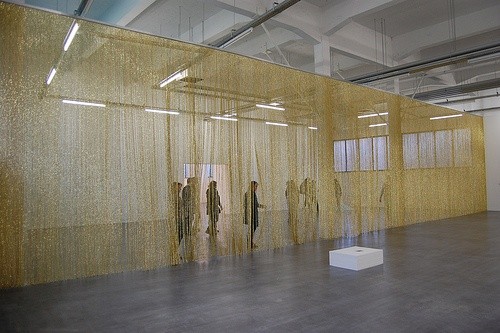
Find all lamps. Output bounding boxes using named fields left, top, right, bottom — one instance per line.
left=159, top=68, right=183, bottom=88
left=61, top=18, right=80, bottom=51
left=46, top=65, right=57, bottom=85
left=62, top=98, right=107, bottom=108
left=255, top=103, right=285, bottom=111
left=145, top=106, right=180, bottom=115
left=218, top=27, right=253, bottom=49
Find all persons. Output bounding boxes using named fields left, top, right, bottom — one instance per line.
left=242, top=181, right=267, bottom=249
left=285, top=180, right=300, bottom=229
left=379, top=175, right=392, bottom=219
left=167, top=182, right=183, bottom=263
left=300, top=177, right=319, bottom=216
left=205, top=180, right=223, bottom=239
left=181, top=177, right=200, bottom=244
left=334, top=178, right=342, bottom=209
